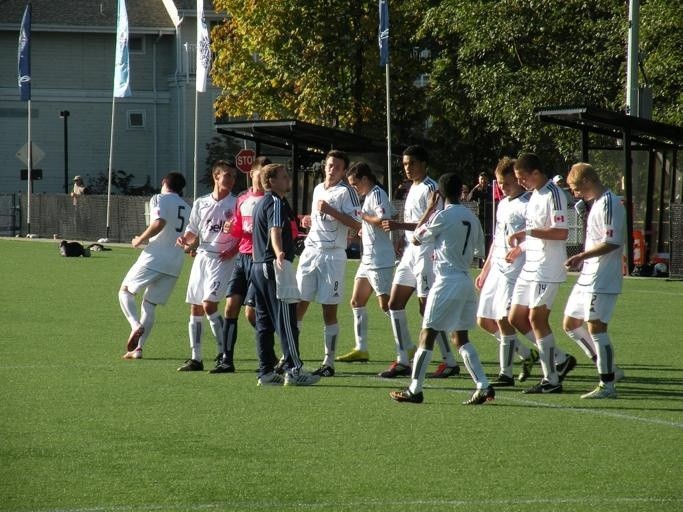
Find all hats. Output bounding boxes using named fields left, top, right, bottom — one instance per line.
left=73, top=176, right=81, bottom=181
left=553, top=175, right=564, bottom=183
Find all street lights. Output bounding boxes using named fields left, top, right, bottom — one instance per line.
left=58, top=109, right=72, bottom=195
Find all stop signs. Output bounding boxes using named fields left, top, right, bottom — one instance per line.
left=235, top=149, right=256, bottom=173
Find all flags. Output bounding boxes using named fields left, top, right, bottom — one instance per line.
left=377, top=1, right=391, bottom=67
left=16, top=4, right=34, bottom=103
left=113, top=0, right=133, bottom=99
left=194, top=1, right=212, bottom=94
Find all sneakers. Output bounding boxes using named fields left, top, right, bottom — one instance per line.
left=335, top=350, right=370, bottom=362
left=380, top=361, right=412, bottom=378
left=210, top=353, right=235, bottom=373
left=177, top=359, right=203, bottom=371
left=389, top=388, right=423, bottom=403
left=255, top=359, right=336, bottom=386
left=463, top=375, right=514, bottom=405
left=580, top=367, right=624, bottom=399
left=518, top=348, right=577, bottom=394
left=124, top=323, right=145, bottom=359
left=427, top=364, right=460, bottom=378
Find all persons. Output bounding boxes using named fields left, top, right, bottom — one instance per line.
left=70, top=174, right=88, bottom=228
left=561, top=163, right=627, bottom=400
left=504, top=153, right=577, bottom=394
left=334, top=161, right=418, bottom=363
left=176, top=158, right=241, bottom=371
left=475, top=158, right=540, bottom=390
left=210, top=156, right=298, bottom=373
left=379, top=145, right=460, bottom=378
left=390, top=174, right=495, bottom=405
left=117, top=171, right=191, bottom=361
left=461, top=171, right=493, bottom=222
left=58, top=240, right=91, bottom=258
left=253, top=163, right=322, bottom=387
left=272, top=150, right=361, bottom=375
